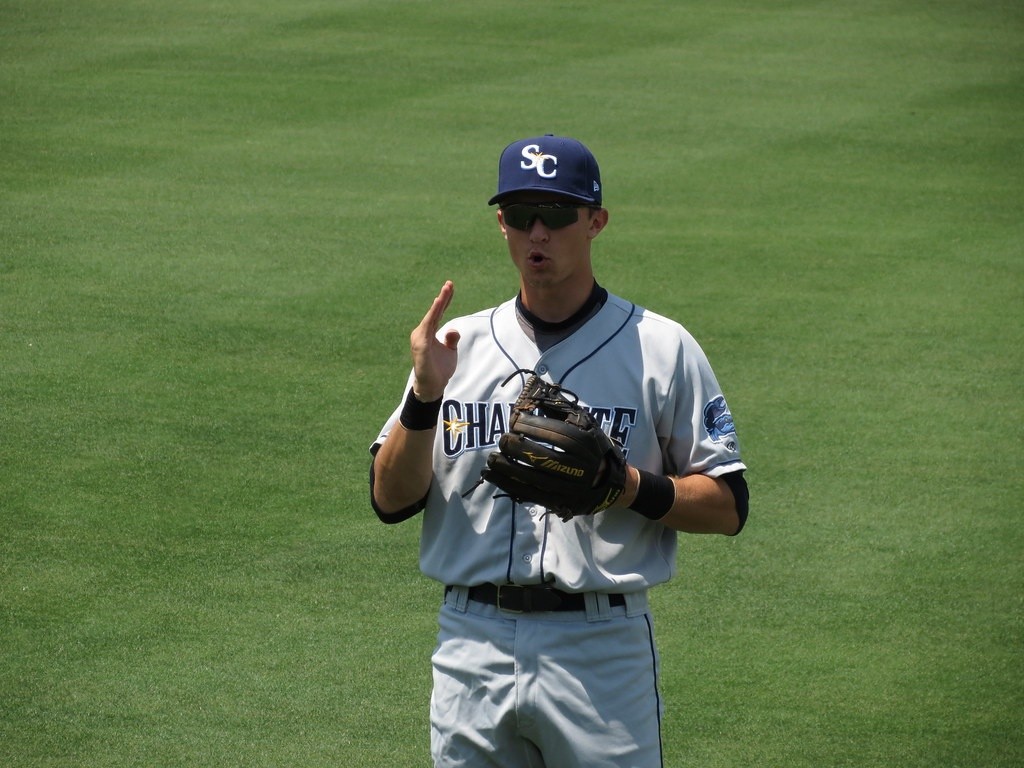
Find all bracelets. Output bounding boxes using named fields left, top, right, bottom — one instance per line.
left=628, top=468, right=676, bottom=522
left=399, top=386, right=444, bottom=431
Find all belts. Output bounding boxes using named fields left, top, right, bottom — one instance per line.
left=446, top=583, right=626, bottom=612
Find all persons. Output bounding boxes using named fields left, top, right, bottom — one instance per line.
left=371, top=136, right=748, bottom=768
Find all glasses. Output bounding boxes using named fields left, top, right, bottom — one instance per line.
left=500, top=200, right=593, bottom=231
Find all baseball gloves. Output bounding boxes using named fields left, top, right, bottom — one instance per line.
left=479, top=373, right=629, bottom=523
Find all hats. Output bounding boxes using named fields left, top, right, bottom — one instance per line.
left=487, top=133, right=602, bottom=207
